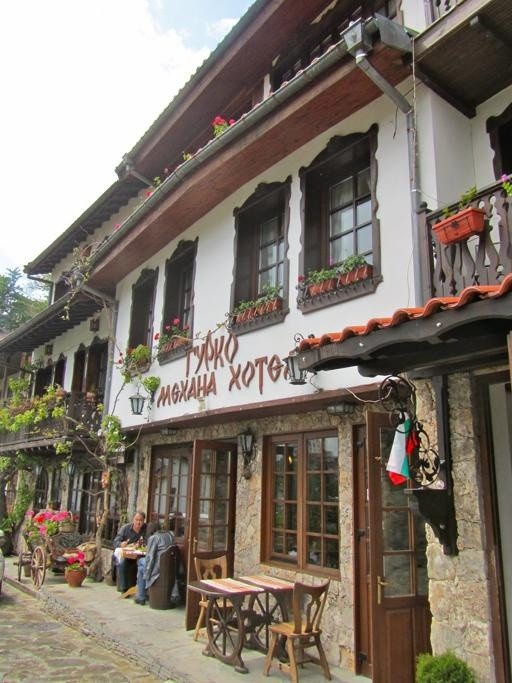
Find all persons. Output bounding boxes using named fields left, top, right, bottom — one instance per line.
left=134, top=522, right=172, bottom=605
left=113, top=513, right=149, bottom=594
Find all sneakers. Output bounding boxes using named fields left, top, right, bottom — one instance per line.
left=135, top=599, right=145, bottom=604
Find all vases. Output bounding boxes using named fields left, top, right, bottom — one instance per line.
left=157, top=335, right=188, bottom=355
left=65, top=569, right=87, bottom=587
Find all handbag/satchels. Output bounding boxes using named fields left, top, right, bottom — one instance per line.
left=170, top=582, right=182, bottom=605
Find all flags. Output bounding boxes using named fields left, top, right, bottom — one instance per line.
left=385, top=410, right=420, bottom=486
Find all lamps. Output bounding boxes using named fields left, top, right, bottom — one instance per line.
left=64, top=461, right=76, bottom=476
left=281, top=332, right=316, bottom=385
left=237, top=426, right=255, bottom=469
left=325, top=400, right=362, bottom=420
left=128, top=380, right=155, bottom=415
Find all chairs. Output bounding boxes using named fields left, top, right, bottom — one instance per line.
left=148, top=545, right=181, bottom=609
left=115, top=562, right=137, bottom=592
left=192, top=555, right=248, bottom=641
left=262, top=576, right=331, bottom=683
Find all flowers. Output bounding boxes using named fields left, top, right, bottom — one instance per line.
left=154, top=318, right=191, bottom=345
left=22, top=510, right=86, bottom=573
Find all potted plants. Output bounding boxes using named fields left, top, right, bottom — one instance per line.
left=431, top=185, right=486, bottom=245
left=304, top=255, right=372, bottom=297
left=231, top=285, right=283, bottom=325
